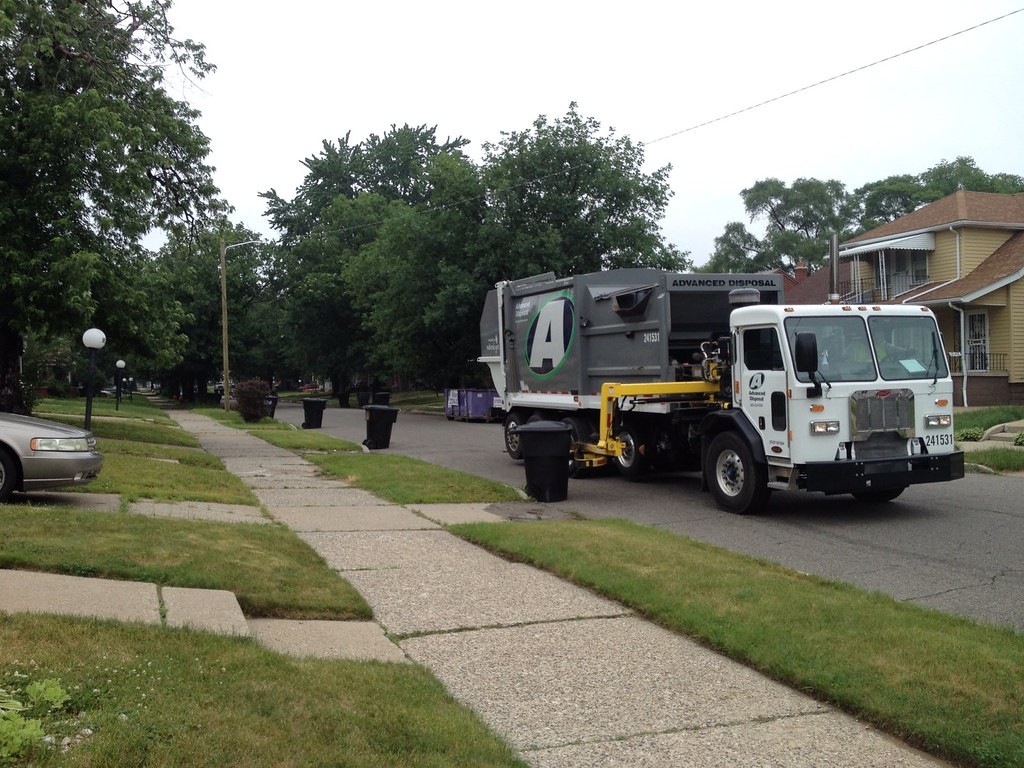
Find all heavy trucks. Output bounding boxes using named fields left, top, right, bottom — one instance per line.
left=476, top=268, right=965, bottom=517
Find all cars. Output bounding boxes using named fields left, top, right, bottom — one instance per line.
left=214, top=375, right=236, bottom=399
left=297, top=384, right=319, bottom=392
left=0, top=411, right=105, bottom=503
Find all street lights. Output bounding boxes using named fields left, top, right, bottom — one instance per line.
left=115, top=359, right=126, bottom=411
left=128, top=377, right=134, bottom=401
left=82, top=328, right=107, bottom=431
left=221, top=241, right=261, bottom=411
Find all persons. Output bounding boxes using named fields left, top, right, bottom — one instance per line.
left=847, top=318, right=912, bottom=363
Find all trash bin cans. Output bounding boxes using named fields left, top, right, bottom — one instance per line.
left=357, top=392, right=371, bottom=409
left=339, top=393, right=349, bottom=408
left=444, top=389, right=502, bottom=423
left=373, top=392, right=390, bottom=405
left=301, top=398, right=327, bottom=429
left=362, top=405, right=399, bottom=450
left=264, top=395, right=278, bottom=419
left=509, top=421, right=573, bottom=503
